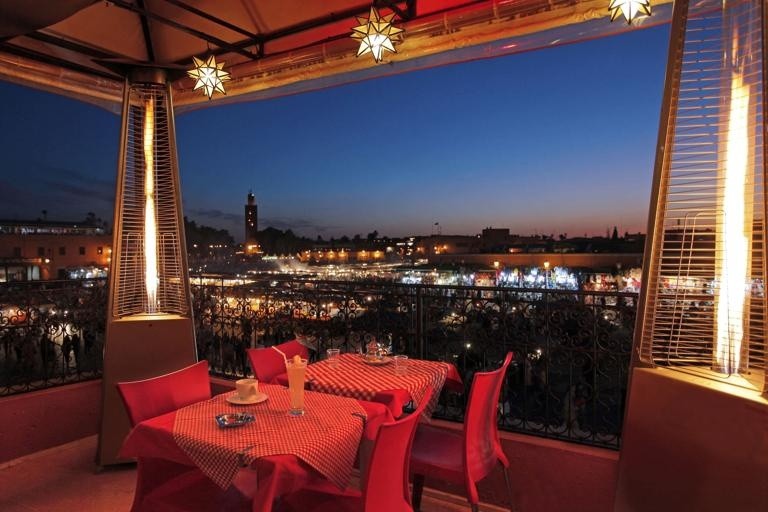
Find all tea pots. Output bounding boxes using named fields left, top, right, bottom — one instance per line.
left=366, top=338, right=388, bottom=362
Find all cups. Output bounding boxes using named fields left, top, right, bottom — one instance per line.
left=284, top=360, right=307, bottom=419
left=396, top=357, right=408, bottom=377
left=327, top=349, right=340, bottom=369
left=237, top=379, right=258, bottom=400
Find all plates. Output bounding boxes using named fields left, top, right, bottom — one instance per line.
left=362, top=356, right=393, bottom=366
left=226, top=393, right=268, bottom=405
left=216, top=413, right=255, bottom=427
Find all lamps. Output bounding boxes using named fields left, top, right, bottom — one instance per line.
left=87, top=60, right=198, bottom=468
left=350, top=7, right=405, bottom=63
left=185, top=55, right=232, bottom=99
left=608, top=1, right=652, bottom=23
left=614, top=0, right=767, bottom=511
